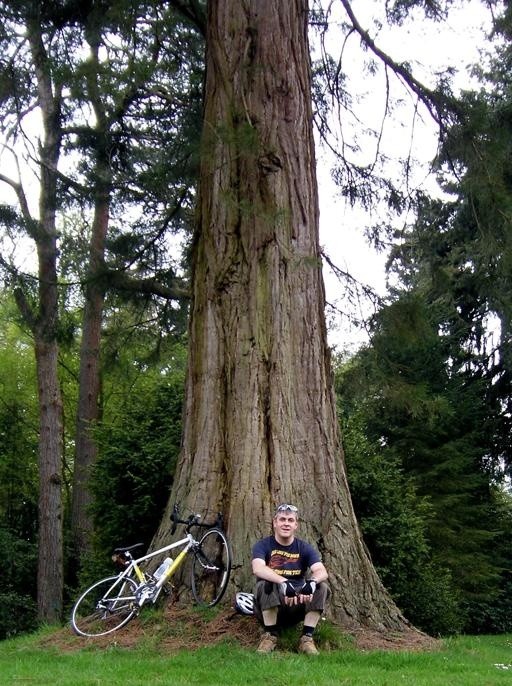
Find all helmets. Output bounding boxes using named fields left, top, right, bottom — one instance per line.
left=234, top=591, right=255, bottom=615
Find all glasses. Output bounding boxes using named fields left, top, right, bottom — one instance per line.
left=275, top=504, right=298, bottom=515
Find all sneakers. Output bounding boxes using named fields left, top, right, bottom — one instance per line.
left=256, top=632, right=277, bottom=653
left=297, top=635, right=319, bottom=655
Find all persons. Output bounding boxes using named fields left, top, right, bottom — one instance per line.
left=250, top=503, right=329, bottom=657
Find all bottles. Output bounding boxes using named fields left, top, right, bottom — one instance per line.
left=154, top=556, right=174, bottom=580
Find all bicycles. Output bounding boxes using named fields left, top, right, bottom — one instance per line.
left=72, top=512, right=234, bottom=640
